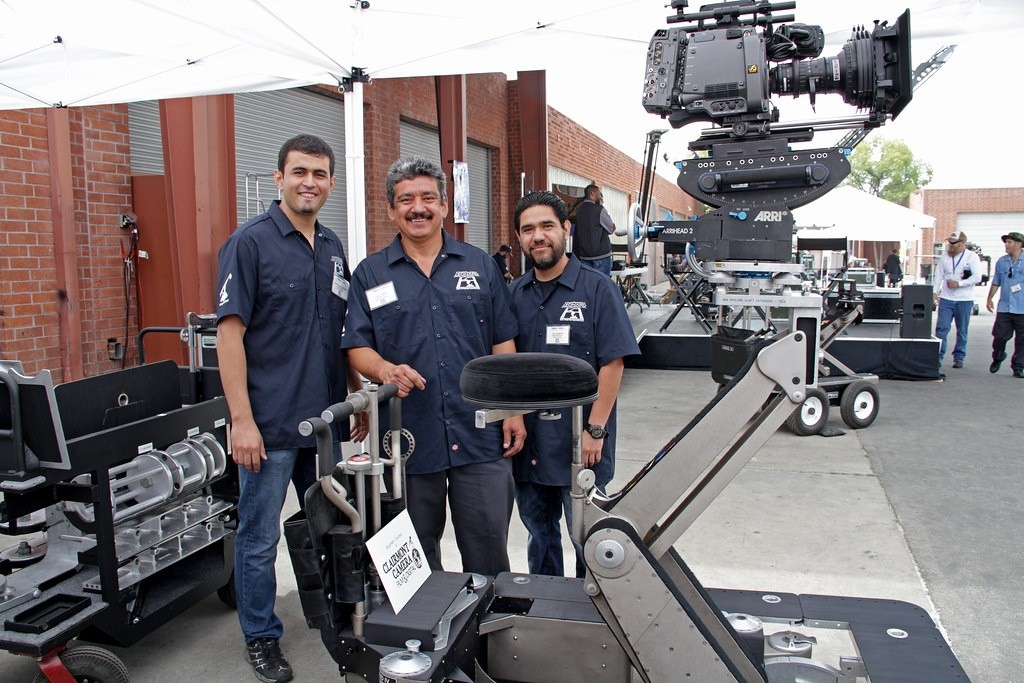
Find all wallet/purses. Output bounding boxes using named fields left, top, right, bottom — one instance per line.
left=961, top=269, right=972, bottom=280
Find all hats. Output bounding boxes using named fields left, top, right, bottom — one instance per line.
left=503, top=244, right=514, bottom=257
left=949, top=232, right=967, bottom=240
left=1002, top=232, right=1024, bottom=247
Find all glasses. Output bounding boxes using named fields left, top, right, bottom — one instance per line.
left=949, top=241, right=961, bottom=244
left=1008, top=267, right=1013, bottom=278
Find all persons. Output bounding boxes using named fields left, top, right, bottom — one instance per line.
left=342, top=157, right=526, bottom=575
left=215, top=133, right=368, bottom=683
left=887, top=249, right=900, bottom=288
left=506, top=189, right=643, bottom=578
left=569, top=184, right=616, bottom=278
left=492, top=244, right=514, bottom=282
left=986, top=233, right=1024, bottom=377
left=933, top=231, right=981, bottom=367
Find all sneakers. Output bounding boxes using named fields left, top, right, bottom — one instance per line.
left=953, top=361, right=963, bottom=368
left=990, top=353, right=1007, bottom=373
left=1013, top=369, right=1024, bottom=378
left=244, top=637, right=293, bottom=683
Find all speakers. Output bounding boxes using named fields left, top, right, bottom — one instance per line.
left=900, top=285, right=933, bottom=339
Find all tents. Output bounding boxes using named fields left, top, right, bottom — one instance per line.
left=795, top=182, right=938, bottom=284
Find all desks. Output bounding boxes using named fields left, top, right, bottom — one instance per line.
left=611, top=266, right=649, bottom=312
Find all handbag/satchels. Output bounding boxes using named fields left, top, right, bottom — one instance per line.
left=883, top=262, right=888, bottom=273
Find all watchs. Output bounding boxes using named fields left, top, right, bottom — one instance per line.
left=586, top=423, right=609, bottom=440
